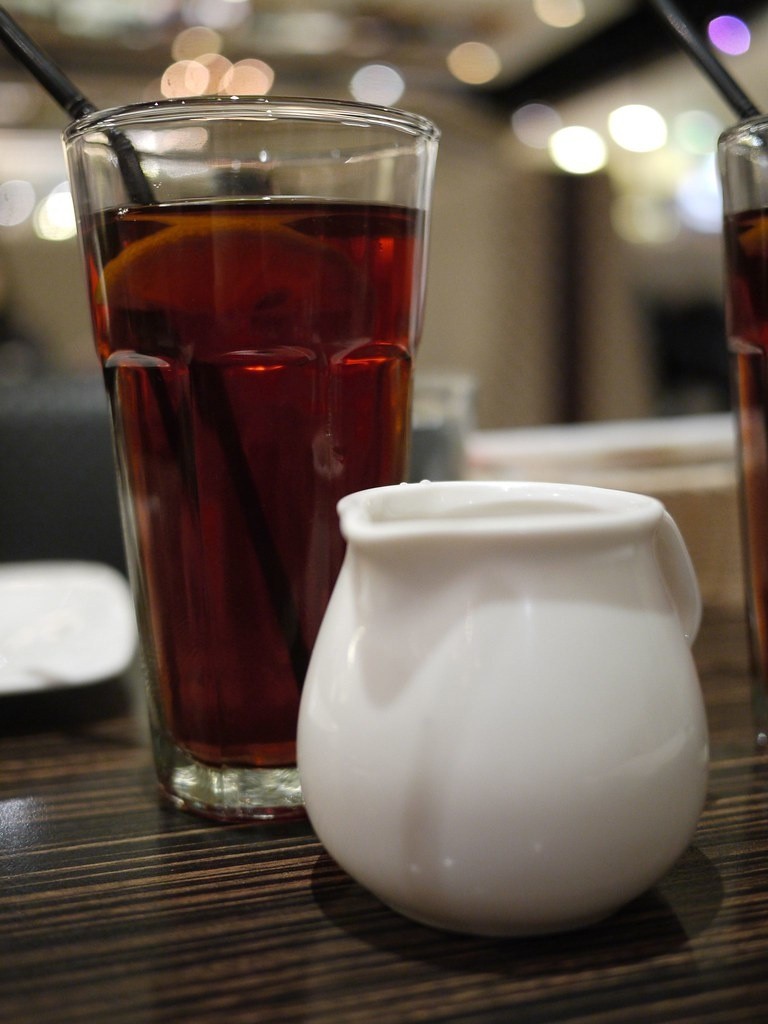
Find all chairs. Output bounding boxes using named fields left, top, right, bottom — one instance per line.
left=2, top=360, right=467, bottom=568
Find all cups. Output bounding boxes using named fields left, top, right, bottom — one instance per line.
left=295, top=479, right=712, bottom=934
left=715, top=114, right=767, bottom=740
left=60, top=96, right=443, bottom=825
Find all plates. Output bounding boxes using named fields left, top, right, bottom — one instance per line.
left=1, top=562, right=138, bottom=695
